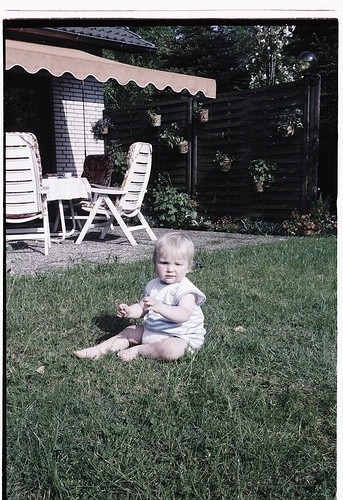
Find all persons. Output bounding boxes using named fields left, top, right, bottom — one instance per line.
left=72, top=231, right=207, bottom=363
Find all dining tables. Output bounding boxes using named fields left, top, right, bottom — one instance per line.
left=41, top=177, right=93, bottom=243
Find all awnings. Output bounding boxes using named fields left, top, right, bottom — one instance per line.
left=5, top=39, right=216, bottom=202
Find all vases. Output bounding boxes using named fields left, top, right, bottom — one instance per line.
left=302, top=229, right=313, bottom=236
left=314, top=229, right=321, bottom=235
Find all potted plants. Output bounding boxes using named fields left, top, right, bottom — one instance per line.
left=91, top=102, right=304, bottom=192
left=153, top=185, right=200, bottom=229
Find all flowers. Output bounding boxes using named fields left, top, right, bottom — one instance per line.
left=314, top=209, right=337, bottom=230
left=282, top=211, right=317, bottom=235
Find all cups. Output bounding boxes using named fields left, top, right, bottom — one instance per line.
left=62, top=172, right=71, bottom=178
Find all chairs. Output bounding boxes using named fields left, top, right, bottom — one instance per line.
left=3, top=132, right=52, bottom=256
left=75, top=142, right=158, bottom=246
left=52, top=154, right=114, bottom=232
